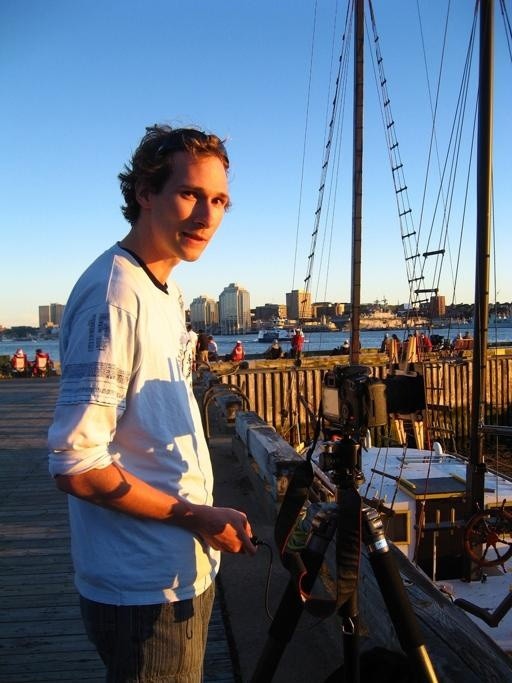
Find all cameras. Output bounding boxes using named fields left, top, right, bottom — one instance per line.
left=320, top=362, right=427, bottom=429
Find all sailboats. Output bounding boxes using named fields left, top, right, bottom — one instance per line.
left=275, top=0, right=512, bottom=661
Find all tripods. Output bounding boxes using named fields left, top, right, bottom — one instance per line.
left=250, top=429, right=439, bottom=683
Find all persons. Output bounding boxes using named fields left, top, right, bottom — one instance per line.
left=11, top=347, right=30, bottom=377
left=185, top=324, right=305, bottom=372
left=47, top=120, right=257, bottom=683
left=379, top=329, right=473, bottom=355
left=31, top=347, right=49, bottom=378
left=341, top=340, right=350, bottom=354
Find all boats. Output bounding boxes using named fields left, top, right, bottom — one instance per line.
left=258, top=317, right=309, bottom=343
